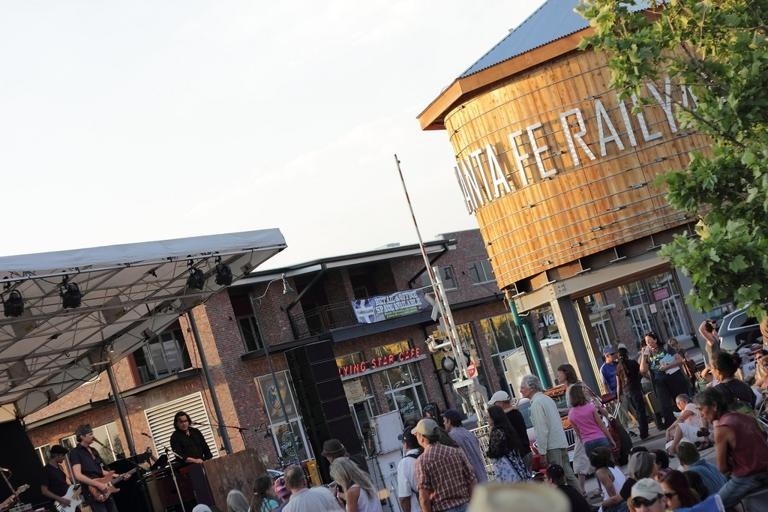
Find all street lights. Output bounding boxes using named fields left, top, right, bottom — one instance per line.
left=248, top=273, right=310, bottom=488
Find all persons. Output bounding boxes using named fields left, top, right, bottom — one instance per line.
left=2, top=317, right=766, bottom=511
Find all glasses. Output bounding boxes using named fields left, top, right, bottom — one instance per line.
left=665, top=492, right=678, bottom=500
left=634, top=497, right=657, bottom=507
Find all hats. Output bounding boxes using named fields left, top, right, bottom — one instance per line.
left=603, top=347, right=615, bottom=355
left=487, top=390, right=511, bottom=405
left=465, top=480, right=572, bottom=512
left=321, top=439, right=345, bottom=457
left=628, top=450, right=658, bottom=479
left=410, top=418, right=439, bottom=436
left=274, top=476, right=292, bottom=499
left=631, top=478, right=664, bottom=502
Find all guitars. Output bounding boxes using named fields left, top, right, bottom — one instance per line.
left=55, top=484, right=86, bottom=511
left=0, top=484, right=30, bottom=511
left=88, top=466, right=137, bottom=502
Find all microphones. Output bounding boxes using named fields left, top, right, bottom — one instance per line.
left=141, top=433, right=152, bottom=439
left=193, top=422, right=201, bottom=425
left=92, top=437, right=104, bottom=447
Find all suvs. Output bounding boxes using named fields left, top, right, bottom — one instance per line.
left=714, top=304, right=761, bottom=352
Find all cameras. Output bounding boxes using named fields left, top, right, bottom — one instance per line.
left=531, top=470, right=543, bottom=480
left=697, top=427, right=710, bottom=437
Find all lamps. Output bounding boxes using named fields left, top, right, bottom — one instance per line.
left=212, top=250, right=235, bottom=286
left=56, top=273, right=83, bottom=313
left=186, top=253, right=206, bottom=288
left=0, top=276, right=28, bottom=317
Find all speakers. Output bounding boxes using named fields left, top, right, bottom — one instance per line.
left=108, top=452, right=154, bottom=512
left=284, top=339, right=371, bottom=486
left=0, top=419, right=57, bottom=512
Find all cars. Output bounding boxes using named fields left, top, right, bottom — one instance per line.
left=513, top=380, right=633, bottom=483
